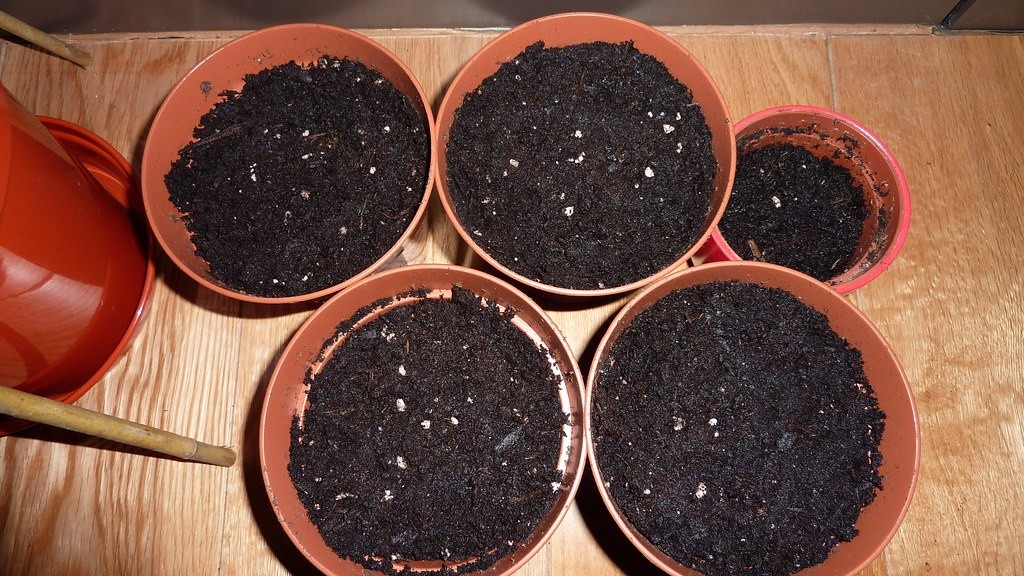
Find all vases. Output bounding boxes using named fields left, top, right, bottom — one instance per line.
left=712, top=103, right=911, bottom=292
left=437, top=11, right=736, bottom=295
left=584, top=260, right=920, bottom=576
left=258, top=264, right=585, bottom=575
left=0, top=85, right=156, bottom=439
left=142, top=23, right=435, bottom=302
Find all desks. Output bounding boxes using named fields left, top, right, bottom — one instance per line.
left=1, top=22, right=1024, bottom=576
